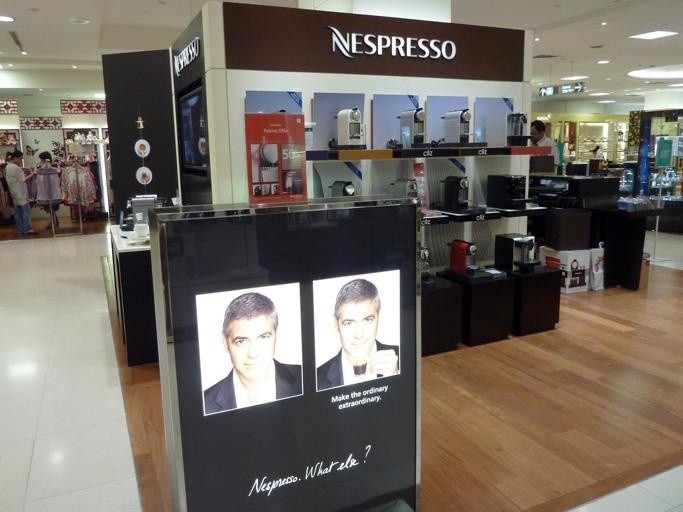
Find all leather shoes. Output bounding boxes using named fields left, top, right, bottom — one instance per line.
left=23, top=231, right=39, bottom=235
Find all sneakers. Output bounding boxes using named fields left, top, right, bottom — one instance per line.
left=18, top=231, right=25, bottom=233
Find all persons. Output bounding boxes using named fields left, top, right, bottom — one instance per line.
left=5, top=151, right=39, bottom=235
left=316, top=278, right=400, bottom=392
left=38, top=151, right=59, bottom=229
left=531, top=120, right=560, bottom=174
left=204, top=292, right=302, bottom=415
left=592, top=146, right=599, bottom=158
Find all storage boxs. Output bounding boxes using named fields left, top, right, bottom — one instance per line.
left=422, top=276, right=461, bottom=357
left=539, top=246, right=591, bottom=295
left=485, top=263, right=563, bottom=338
left=436, top=267, right=514, bottom=347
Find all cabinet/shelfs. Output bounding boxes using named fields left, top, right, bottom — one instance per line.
left=578, top=121, right=629, bottom=163
left=607, top=167, right=683, bottom=234
left=44, top=159, right=84, bottom=238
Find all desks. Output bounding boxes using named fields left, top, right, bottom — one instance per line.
left=110, top=223, right=158, bottom=367
left=33, top=159, right=61, bottom=175
